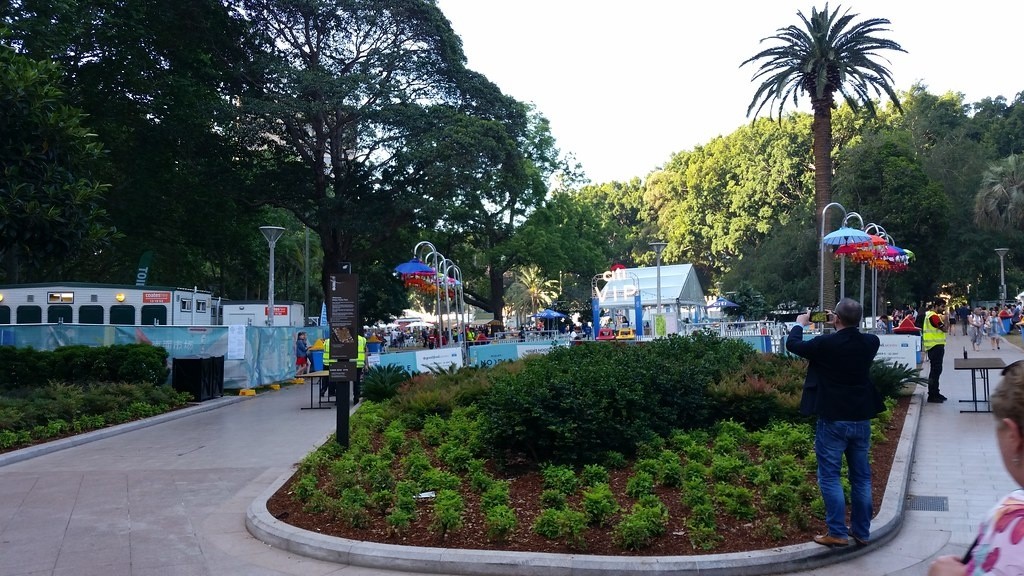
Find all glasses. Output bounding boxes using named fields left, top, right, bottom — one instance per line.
left=831, top=309, right=840, bottom=319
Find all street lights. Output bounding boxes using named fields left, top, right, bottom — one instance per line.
left=994, top=248, right=1011, bottom=310
left=258, top=226, right=287, bottom=328
left=648, top=241, right=668, bottom=313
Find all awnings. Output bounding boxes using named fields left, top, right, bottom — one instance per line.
left=364, top=318, right=434, bottom=333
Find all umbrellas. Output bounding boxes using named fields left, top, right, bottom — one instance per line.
left=530, top=308, right=569, bottom=340
left=704, top=297, right=742, bottom=323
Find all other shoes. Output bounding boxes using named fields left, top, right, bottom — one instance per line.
left=846, top=527, right=869, bottom=545
left=936, top=394, right=947, bottom=400
left=998, top=347, right=1000, bottom=349
left=927, top=396, right=943, bottom=403
left=320, top=389, right=324, bottom=397
left=814, top=533, right=849, bottom=546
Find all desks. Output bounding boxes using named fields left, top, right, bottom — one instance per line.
left=294, top=370, right=337, bottom=409
left=954, top=358, right=1006, bottom=413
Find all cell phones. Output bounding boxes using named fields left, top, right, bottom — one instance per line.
left=809, top=312, right=829, bottom=322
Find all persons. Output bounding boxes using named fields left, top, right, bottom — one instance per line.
left=923, top=298, right=949, bottom=404
left=294, top=330, right=311, bottom=375
left=782, top=298, right=888, bottom=550
left=926, top=360, right=1024, bottom=576
left=874, top=295, right=1024, bottom=355
left=683, top=316, right=770, bottom=332
left=362, top=320, right=546, bottom=356
left=562, top=314, right=650, bottom=344
left=317, top=335, right=368, bottom=407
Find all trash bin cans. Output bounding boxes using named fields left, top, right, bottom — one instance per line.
left=366, top=342, right=381, bottom=355
left=309, top=349, right=324, bottom=372
